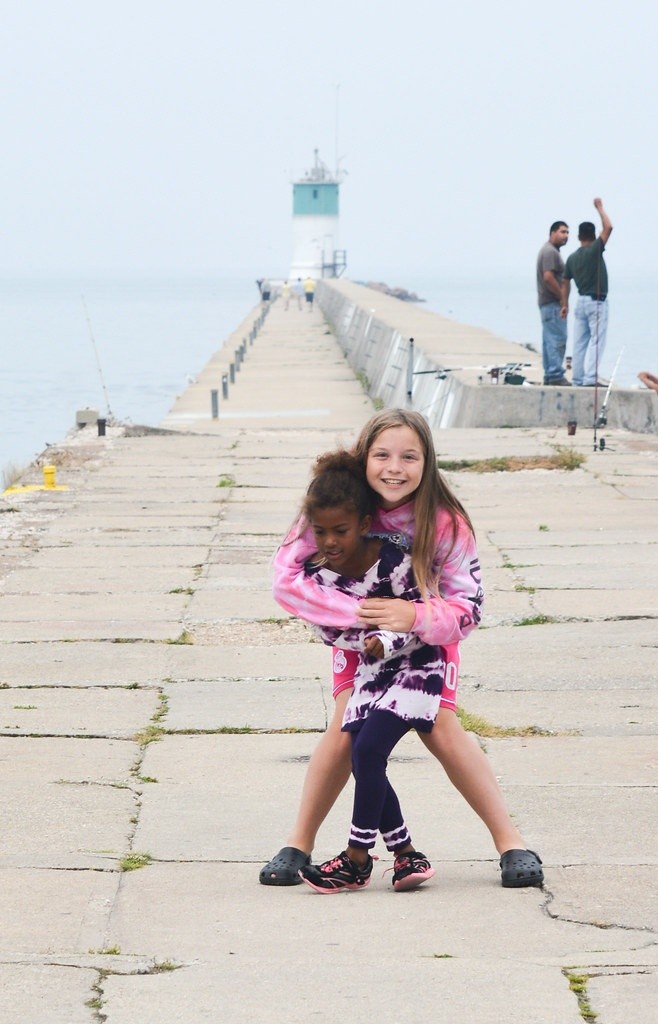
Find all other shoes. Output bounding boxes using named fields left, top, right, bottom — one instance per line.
left=585, top=383, right=609, bottom=388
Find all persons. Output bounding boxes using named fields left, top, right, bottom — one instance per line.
left=296, top=277, right=315, bottom=310
left=537, top=221, right=571, bottom=386
left=261, top=279, right=271, bottom=300
left=283, top=282, right=290, bottom=310
left=260, top=408, right=543, bottom=892
left=561, top=198, right=612, bottom=387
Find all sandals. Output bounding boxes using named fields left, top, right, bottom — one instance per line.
left=260, top=848, right=311, bottom=885
left=501, top=845, right=548, bottom=888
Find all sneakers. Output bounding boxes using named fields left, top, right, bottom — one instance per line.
left=300, top=849, right=378, bottom=895
left=391, top=851, right=438, bottom=893
left=548, top=377, right=572, bottom=386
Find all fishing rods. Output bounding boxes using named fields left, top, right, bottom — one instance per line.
left=597, top=343, right=627, bottom=427
left=412, top=361, right=543, bottom=377
